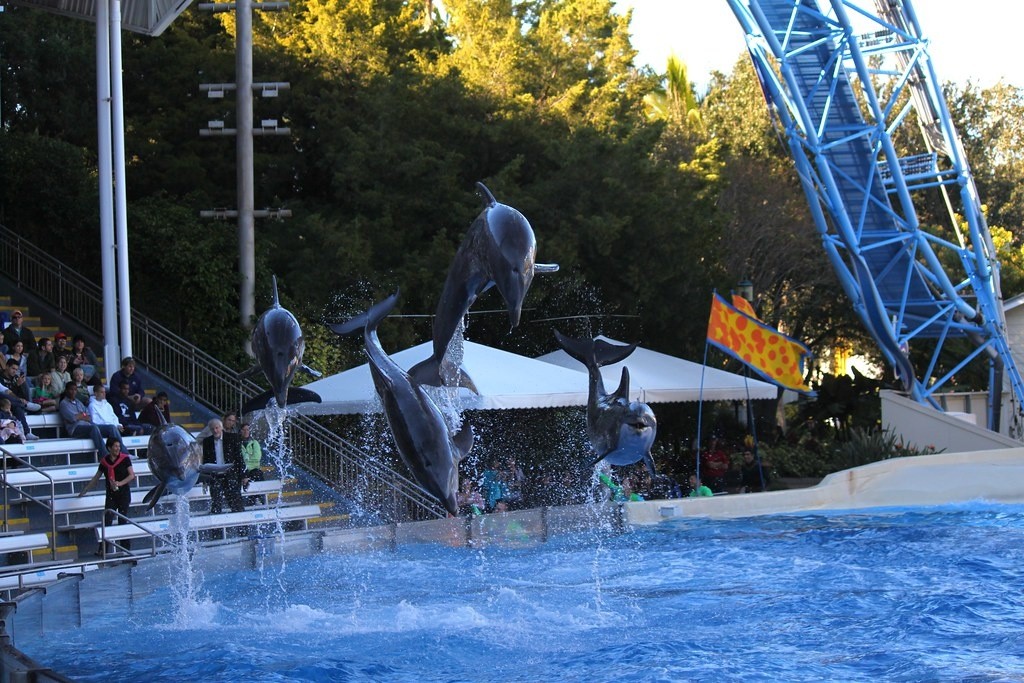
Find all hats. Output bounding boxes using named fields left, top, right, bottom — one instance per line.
left=55, top=333, right=67, bottom=338
left=11, top=309, right=23, bottom=315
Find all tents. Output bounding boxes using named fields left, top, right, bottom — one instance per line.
left=535, top=333, right=777, bottom=402
left=284, top=339, right=640, bottom=415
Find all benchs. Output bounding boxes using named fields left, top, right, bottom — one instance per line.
left=94, top=504, right=341, bottom=558
left=0, top=562, right=99, bottom=601
left=0, top=533, right=73, bottom=572
left=0, top=459, right=205, bottom=507
left=47, top=479, right=302, bottom=533
left=0, top=432, right=204, bottom=474
left=0, top=412, right=141, bottom=443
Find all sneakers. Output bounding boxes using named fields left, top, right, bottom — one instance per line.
left=24, top=401, right=42, bottom=413
left=25, top=433, right=39, bottom=441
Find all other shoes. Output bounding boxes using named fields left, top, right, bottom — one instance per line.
left=94, top=550, right=105, bottom=556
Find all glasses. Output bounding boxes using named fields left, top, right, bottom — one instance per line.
left=13, top=315, right=23, bottom=319
left=58, top=339, right=67, bottom=342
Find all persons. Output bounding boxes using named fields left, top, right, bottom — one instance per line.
left=77, top=436, right=135, bottom=556
left=201, top=419, right=252, bottom=539
left=0, top=308, right=266, bottom=505
left=457, top=415, right=824, bottom=514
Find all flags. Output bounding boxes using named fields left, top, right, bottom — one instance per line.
left=709, top=293, right=813, bottom=394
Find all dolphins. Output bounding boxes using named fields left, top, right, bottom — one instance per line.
left=142, top=402, right=235, bottom=512
left=324, top=285, right=474, bottom=517
left=404, top=180, right=561, bottom=396
left=233, top=275, right=323, bottom=416
left=553, top=328, right=658, bottom=478
